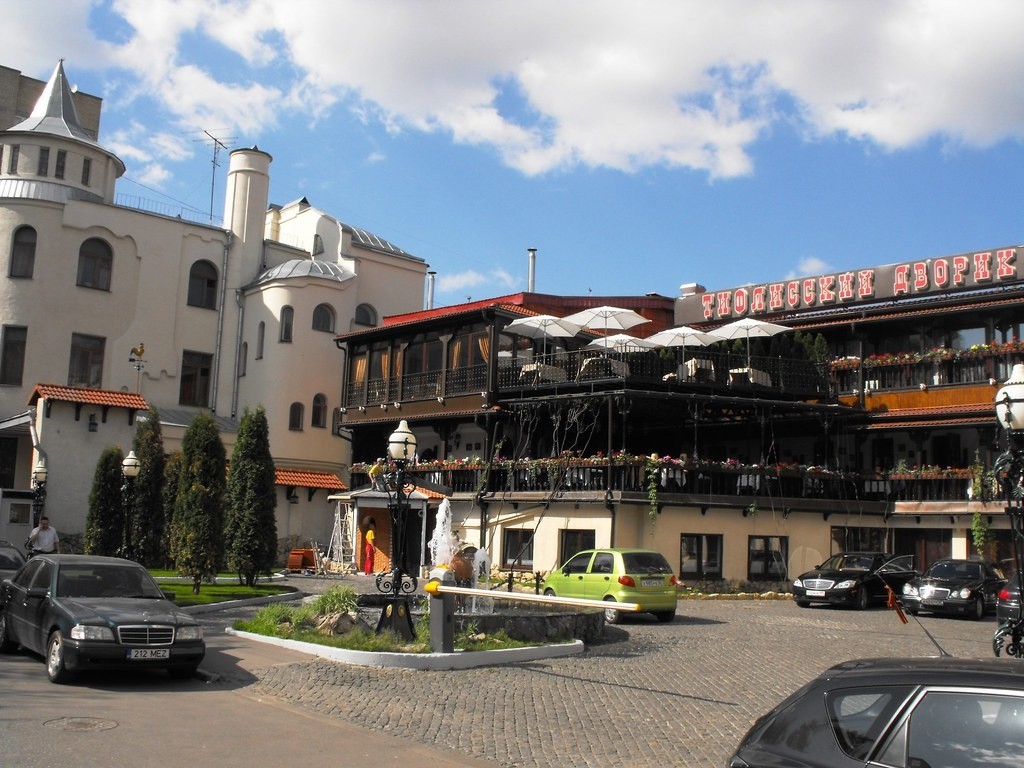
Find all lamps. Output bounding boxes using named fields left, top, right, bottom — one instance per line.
left=359, top=406, right=365, bottom=412
left=989, top=378, right=996, bottom=386
left=438, top=396, right=444, bottom=403
left=341, top=408, right=346, bottom=413
left=381, top=404, right=387, bottom=410
left=482, top=392, right=488, bottom=398
left=920, top=383, right=927, bottom=391
left=864, top=389, right=870, bottom=395
left=394, top=402, right=400, bottom=408
left=852, top=388, right=858, bottom=396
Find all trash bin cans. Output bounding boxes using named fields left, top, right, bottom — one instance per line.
left=292, top=549, right=319, bottom=568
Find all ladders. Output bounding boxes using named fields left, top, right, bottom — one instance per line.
left=327, top=501, right=358, bottom=574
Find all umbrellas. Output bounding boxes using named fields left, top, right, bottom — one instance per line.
left=503, top=313, right=583, bottom=365
left=587, top=333, right=656, bottom=362
left=644, top=325, right=720, bottom=381
left=562, top=305, right=653, bottom=359
left=706, top=318, right=794, bottom=368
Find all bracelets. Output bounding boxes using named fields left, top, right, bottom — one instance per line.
left=57, top=551, right=60, bottom=553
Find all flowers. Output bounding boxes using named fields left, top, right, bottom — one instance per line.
left=352, top=453, right=998, bottom=558
left=827, top=337, right=1024, bottom=368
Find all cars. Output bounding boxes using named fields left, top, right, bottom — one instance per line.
left=749, top=549, right=787, bottom=580
left=727, top=657, right=1024, bottom=768
left=543, top=548, right=678, bottom=623
left=996, top=571, right=1023, bottom=626
left=682, top=550, right=721, bottom=574
left=791, top=552, right=918, bottom=610
left=902, top=559, right=1006, bottom=621
left=0, top=554, right=205, bottom=683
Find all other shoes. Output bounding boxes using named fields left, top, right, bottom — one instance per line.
left=367, top=573, right=375, bottom=577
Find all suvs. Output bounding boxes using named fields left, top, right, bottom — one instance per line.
left=0, top=541, right=30, bottom=596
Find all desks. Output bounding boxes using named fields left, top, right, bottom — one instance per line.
left=579, top=358, right=630, bottom=380
left=729, top=368, right=772, bottom=388
left=566, top=467, right=602, bottom=489
left=519, top=364, right=567, bottom=383
left=654, top=467, right=686, bottom=493
left=676, top=359, right=716, bottom=383
left=737, top=475, right=778, bottom=496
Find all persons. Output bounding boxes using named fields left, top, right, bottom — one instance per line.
left=29, top=517, right=61, bottom=557
left=363, top=516, right=376, bottom=575
left=368, top=458, right=384, bottom=490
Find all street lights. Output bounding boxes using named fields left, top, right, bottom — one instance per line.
left=375, top=420, right=422, bottom=643
left=991, top=362, right=1024, bottom=659
left=118, top=450, right=143, bottom=563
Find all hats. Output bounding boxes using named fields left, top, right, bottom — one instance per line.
left=377, top=458, right=385, bottom=462
